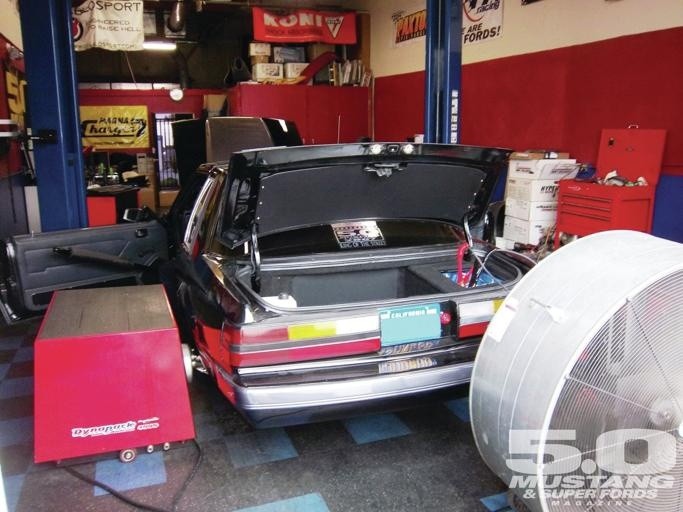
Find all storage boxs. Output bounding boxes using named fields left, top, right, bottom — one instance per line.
left=308, top=44, right=334, bottom=61
left=285, top=62, right=314, bottom=86
left=250, top=42, right=271, bottom=57
left=251, top=63, right=284, bottom=83
left=274, top=45, right=305, bottom=64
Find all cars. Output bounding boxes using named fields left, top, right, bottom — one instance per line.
left=0, top=116, right=525, bottom=433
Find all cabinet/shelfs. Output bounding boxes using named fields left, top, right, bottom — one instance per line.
left=228, top=83, right=372, bottom=145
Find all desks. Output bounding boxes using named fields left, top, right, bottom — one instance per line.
left=85, top=182, right=142, bottom=225
